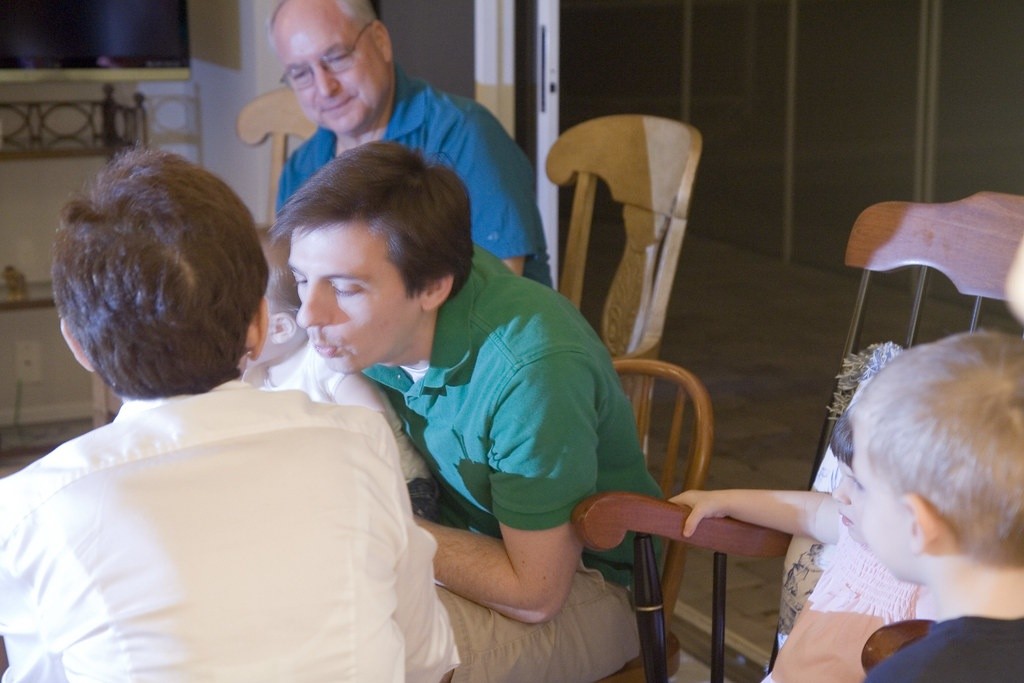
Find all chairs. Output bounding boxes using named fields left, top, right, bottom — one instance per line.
left=236, top=85, right=1024, bottom=683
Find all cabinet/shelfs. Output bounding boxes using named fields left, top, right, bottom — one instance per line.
left=0, top=81, right=151, bottom=479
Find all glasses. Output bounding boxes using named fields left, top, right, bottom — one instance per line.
left=280, top=21, right=374, bottom=91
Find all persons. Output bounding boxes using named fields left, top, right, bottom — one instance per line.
left=240, top=0, right=553, bottom=515
left=0, top=149, right=462, bottom=683
left=849, top=332, right=1024, bottom=683
left=271, top=141, right=667, bottom=683
left=669, top=403, right=936, bottom=683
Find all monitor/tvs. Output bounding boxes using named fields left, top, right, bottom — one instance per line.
left=0, top=0, right=192, bottom=69
